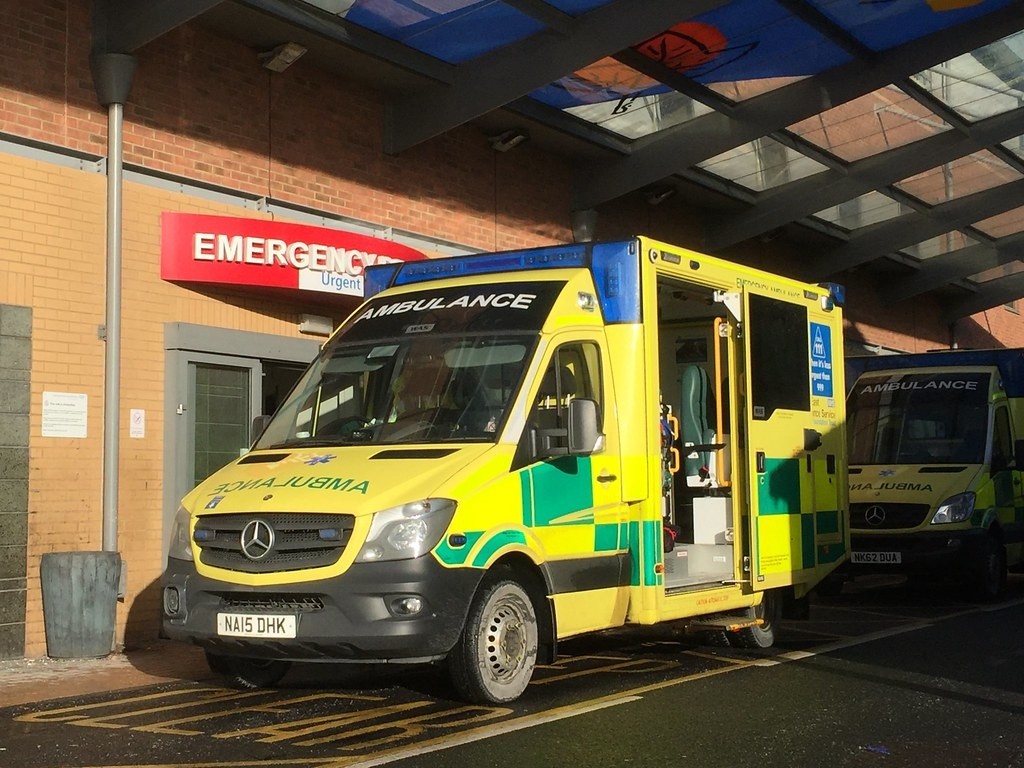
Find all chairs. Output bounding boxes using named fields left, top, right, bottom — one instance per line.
left=681, top=366, right=730, bottom=489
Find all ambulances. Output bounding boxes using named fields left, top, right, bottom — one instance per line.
left=835, top=347, right=1024, bottom=604
left=160, top=236, right=851, bottom=708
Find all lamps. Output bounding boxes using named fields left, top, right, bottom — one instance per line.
left=646, top=186, right=677, bottom=205
left=262, top=40, right=308, bottom=74
left=760, top=228, right=788, bottom=243
left=489, top=127, right=530, bottom=152
